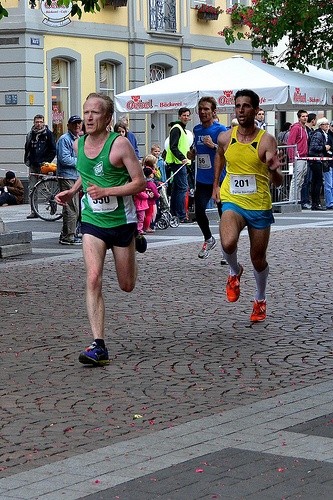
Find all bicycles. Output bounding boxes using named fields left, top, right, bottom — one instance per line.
left=26, top=171, right=65, bottom=222
left=150, top=196, right=181, bottom=231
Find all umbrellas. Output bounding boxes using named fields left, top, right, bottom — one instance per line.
left=114, top=54, right=333, bottom=116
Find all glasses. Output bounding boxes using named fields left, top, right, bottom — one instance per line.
left=322, top=123, right=329, bottom=125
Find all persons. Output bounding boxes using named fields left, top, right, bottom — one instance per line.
left=55, top=92, right=149, bottom=366
left=211, top=88, right=287, bottom=323
left=23, top=114, right=57, bottom=219
left=55, top=106, right=333, bottom=246
left=186, top=96, right=230, bottom=266
left=0, top=171, right=24, bottom=207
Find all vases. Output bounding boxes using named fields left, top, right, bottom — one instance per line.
left=198, top=11, right=218, bottom=20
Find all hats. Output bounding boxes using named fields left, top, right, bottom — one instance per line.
left=68, top=115, right=82, bottom=124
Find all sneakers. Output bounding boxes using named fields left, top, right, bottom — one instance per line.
left=136, top=234, right=147, bottom=253
left=221, top=256, right=229, bottom=265
left=198, top=236, right=217, bottom=259
left=250, top=297, right=267, bottom=322
left=78, top=342, right=111, bottom=365
left=226, top=263, right=243, bottom=302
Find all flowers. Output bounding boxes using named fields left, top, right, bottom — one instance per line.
left=195, top=4, right=224, bottom=14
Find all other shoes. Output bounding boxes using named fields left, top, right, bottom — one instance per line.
left=27, top=213, right=39, bottom=218
left=59, top=234, right=83, bottom=245
left=311, top=201, right=327, bottom=210
left=146, top=227, right=155, bottom=232
left=50, top=198, right=57, bottom=215
left=179, top=217, right=194, bottom=223
